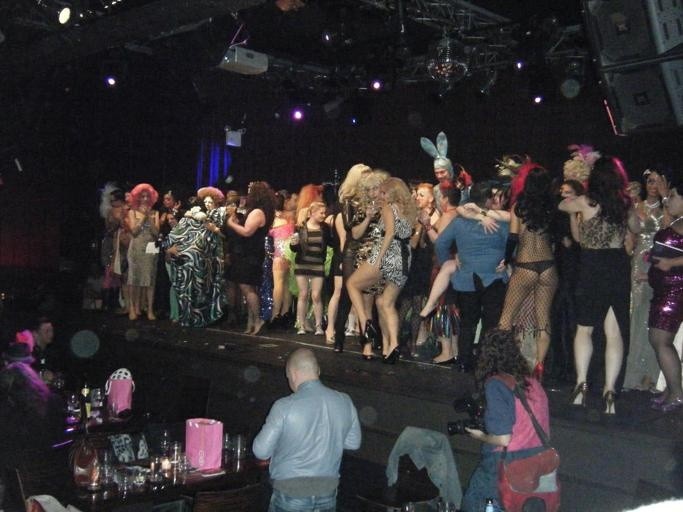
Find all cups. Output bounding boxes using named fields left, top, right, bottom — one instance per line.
left=401, top=500, right=415, bottom=512
left=220, top=432, right=248, bottom=468
left=438, top=502, right=455, bottom=512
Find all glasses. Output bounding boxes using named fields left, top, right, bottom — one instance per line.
left=110, top=199, right=117, bottom=202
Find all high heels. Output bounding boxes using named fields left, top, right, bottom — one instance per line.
left=146, top=313, right=157, bottom=320
left=416, top=307, right=437, bottom=321
left=600, top=390, right=618, bottom=423
left=531, top=361, right=544, bottom=384
left=128, top=309, right=139, bottom=320
left=567, top=382, right=589, bottom=407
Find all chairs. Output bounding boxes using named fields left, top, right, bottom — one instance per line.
left=7, top=325, right=459, bottom=512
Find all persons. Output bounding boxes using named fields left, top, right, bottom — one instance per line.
left=252, top=348, right=362, bottom=512
left=461, top=328, right=548, bottom=512
left=100, top=132, right=683, bottom=417
left=0, top=315, right=69, bottom=471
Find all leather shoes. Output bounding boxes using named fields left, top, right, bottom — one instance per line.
left=663, top=400, right=683, bottom=414
left=649, top=390, right=669, bottom=410
left=244, top=319, right=462, bottom=366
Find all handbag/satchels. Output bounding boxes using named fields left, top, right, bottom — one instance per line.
left=499, top=384, right=560, bottom=512
left=105, top=378, right=136, bottom=416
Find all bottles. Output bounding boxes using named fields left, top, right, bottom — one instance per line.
left=485, top=498, right=494, bottom=511
left=39, top=357, right=104, bottom=424
left=87, top=428, right=189, bottom=502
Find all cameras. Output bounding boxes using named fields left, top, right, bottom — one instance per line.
left=447, top=397, right=486, bottom=436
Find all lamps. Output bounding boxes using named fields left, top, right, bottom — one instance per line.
left=320, top=20, right=357, bottom=47
left=36, top=0, right=72, bottom=25
left=425, top=36, right=471, bottom=99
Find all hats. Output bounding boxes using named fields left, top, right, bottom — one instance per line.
left=6, top=342, right=36, bottom=364
